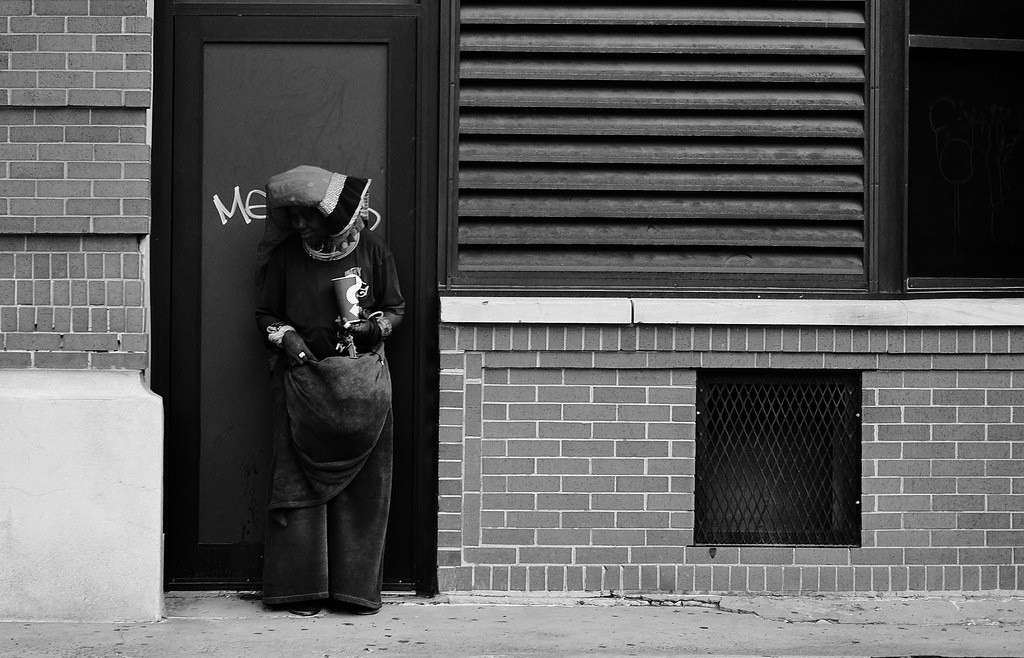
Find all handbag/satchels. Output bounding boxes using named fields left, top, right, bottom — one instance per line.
left=280, top=341, right=393, bottom=473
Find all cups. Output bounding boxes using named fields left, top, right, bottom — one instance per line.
left=330, top=276, right=361, bottom=323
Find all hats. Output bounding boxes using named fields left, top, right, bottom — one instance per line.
left=257, top=164, right=373, bottom=264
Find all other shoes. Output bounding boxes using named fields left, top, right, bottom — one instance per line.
left=334, top=599, right=382, bottom=616
left=288, top=598, right=323, bottom=617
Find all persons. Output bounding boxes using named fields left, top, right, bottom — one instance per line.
left=253, top=165, right=403, bottom=616
